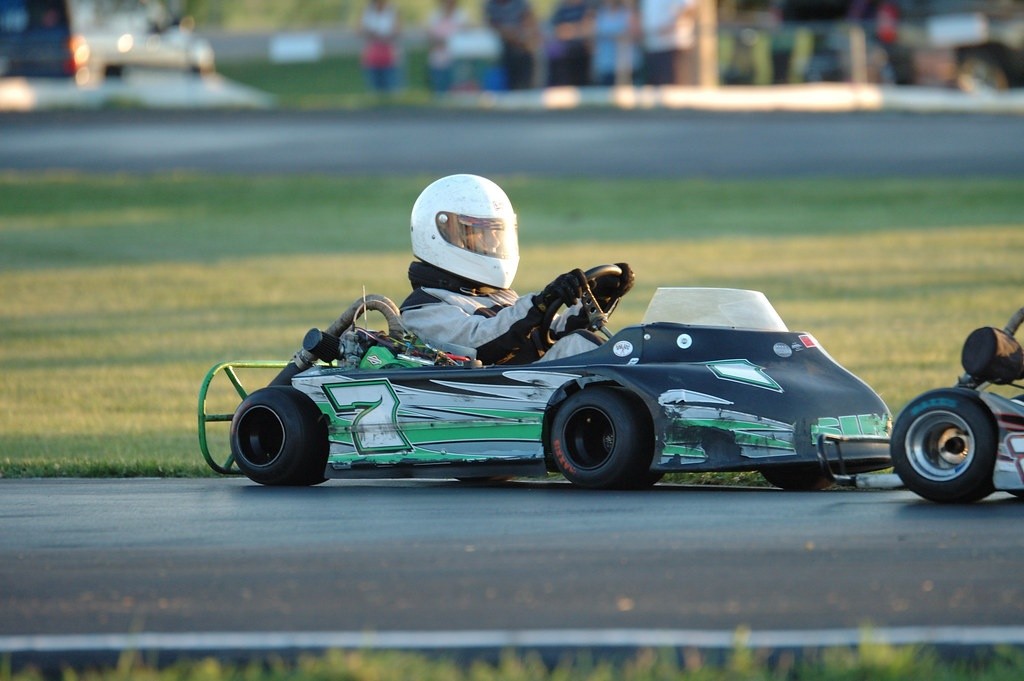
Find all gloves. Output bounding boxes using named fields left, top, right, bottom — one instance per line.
left=595, top=262, right=635, bottom=303
left=532, top=268, right=597, bottom=316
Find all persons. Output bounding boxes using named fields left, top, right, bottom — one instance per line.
left=399, top=174, right=634, bottom=364
left=363, top=0, right=694, bottom=86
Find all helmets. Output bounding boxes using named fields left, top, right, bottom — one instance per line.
left=411, top=174, right=520, bottom=289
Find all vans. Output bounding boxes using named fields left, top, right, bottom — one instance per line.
left=0, top=0, right=223, bottom=86
